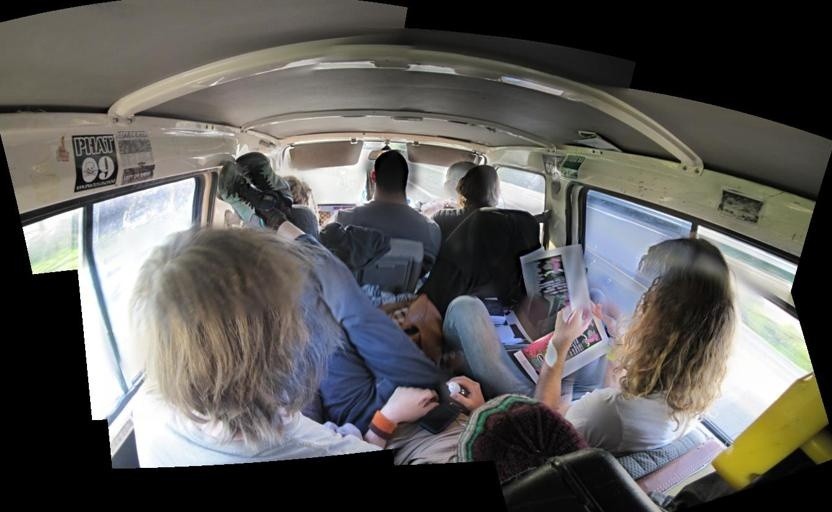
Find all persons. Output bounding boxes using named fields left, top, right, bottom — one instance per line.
left=442, top=268, right=737, bottom=457
left=131, top=223, right=385, bottom=469
left=430, top=164, right=501, bottom=240
left=282, top=175, right=309, bottom=205
left=217, top=153, right=590, bottom=485
left=633, top=238, right=730, bottom=290
left=331, top=152, right=442, bottom=279
left=422, top=160, right=475, bottom=216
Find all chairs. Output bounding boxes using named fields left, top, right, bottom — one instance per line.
left=288, top=204, right=541, bottom=307
left=616, top=418, right=725, bottom=496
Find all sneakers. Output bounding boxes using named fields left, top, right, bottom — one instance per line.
left=217, top=153, right=293, bottom=231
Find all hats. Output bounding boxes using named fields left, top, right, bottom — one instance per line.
left=455, top=393, right=590, bottom=486
left=458, top=165, right=500, bottom=206
left=444, top=161, right=477, bottom=192
